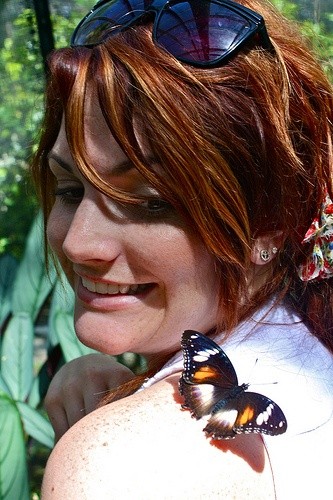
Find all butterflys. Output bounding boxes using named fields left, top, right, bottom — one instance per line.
left=178, top=329, right=288, bottom=441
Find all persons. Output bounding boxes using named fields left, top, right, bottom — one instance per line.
left=31, top=0, right=332, bottom=500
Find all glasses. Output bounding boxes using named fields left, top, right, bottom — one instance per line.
left=71, top=0, right=284, bottom=77
